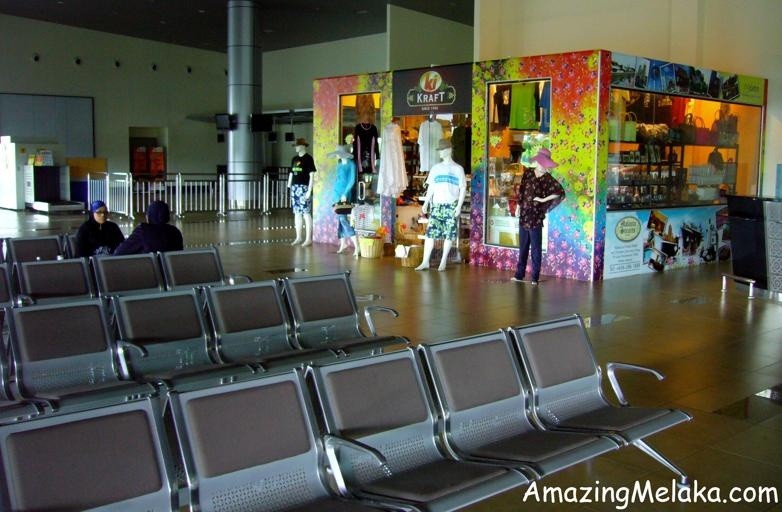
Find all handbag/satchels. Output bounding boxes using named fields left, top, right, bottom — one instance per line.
left=331, top=202, right=355, bottom=214
left=609, top=109, right=739, bottom=147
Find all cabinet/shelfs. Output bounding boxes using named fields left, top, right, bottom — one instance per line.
left=724, top=191, right=774, bottom=290
left=606, top=137, right=740, bottom=211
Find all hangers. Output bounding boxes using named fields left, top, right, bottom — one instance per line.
left=391, top=115, right=400, bottom=126
left=427, top=112, right=436, bottom=122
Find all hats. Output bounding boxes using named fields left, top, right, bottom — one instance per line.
left=291, top=138, right=560, bottom=169
left=91, top=200, right=105, bottom=213
left=147, top=200, right=170, bottom=225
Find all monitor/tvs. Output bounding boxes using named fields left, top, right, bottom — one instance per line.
left=215, top=113, right=232, bottom=130
left=217, top=134, right=224, bottom=143
left=267, top=132, right=277, bottom=141
left=285, top=132, right=294, bottom=143
left=250, top=112, right=274, bottom=132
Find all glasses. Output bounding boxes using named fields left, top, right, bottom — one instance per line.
left=96, top=210, right=108, bottom=215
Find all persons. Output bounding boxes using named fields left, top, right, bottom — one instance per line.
left=326, top=145, right=360, bottom=257
left=510, top=148, right=566, bottom=286
left=74, top=201, right=125, bottom=259
left=286, top=137, right=317, bottom=247
left=113, top=200, right=183, bottom=256
left=414, top=138, right=467, bottom=271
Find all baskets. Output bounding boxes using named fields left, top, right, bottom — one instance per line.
left=400, top=245, right=424, bottom=268
left=358, top=236, right=385, bottom=259
